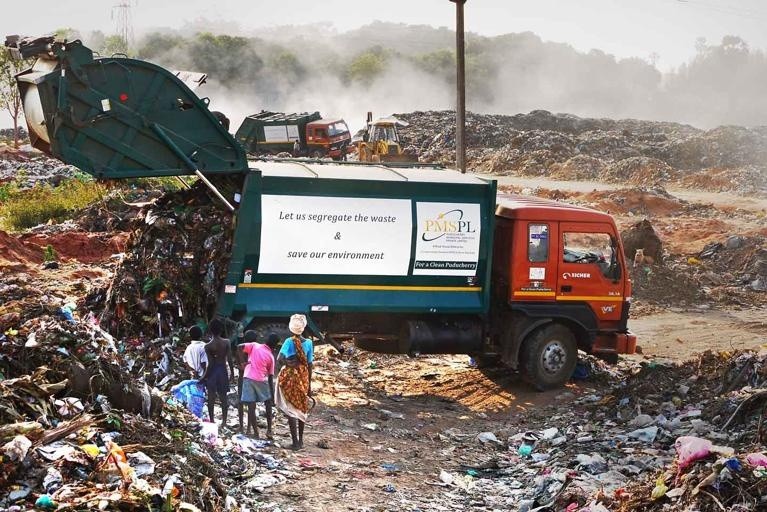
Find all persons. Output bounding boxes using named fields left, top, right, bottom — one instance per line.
left=273, top=314, right=314, bottom=452
left=204, top=321, right=234, bottom=424
left=183, top=325, right=207, bottom=382
left=293, top=139, right=300, bottom=157
left=236, top=332, right=281, bottom=440
left=235, top=329, right=254, bottom=436
left=363, top=130, right=369, bottom=142
left=338, top=140, right=347, bottom=161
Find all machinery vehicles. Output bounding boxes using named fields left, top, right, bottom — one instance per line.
left=15, top=37, right=637, bottom=391
left=352, top=111, right=417, bottom=168
left=234, top=110, right=355, bottom=162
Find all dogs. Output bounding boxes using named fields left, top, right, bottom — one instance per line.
left=633, top=248, right=654, bottom=268
